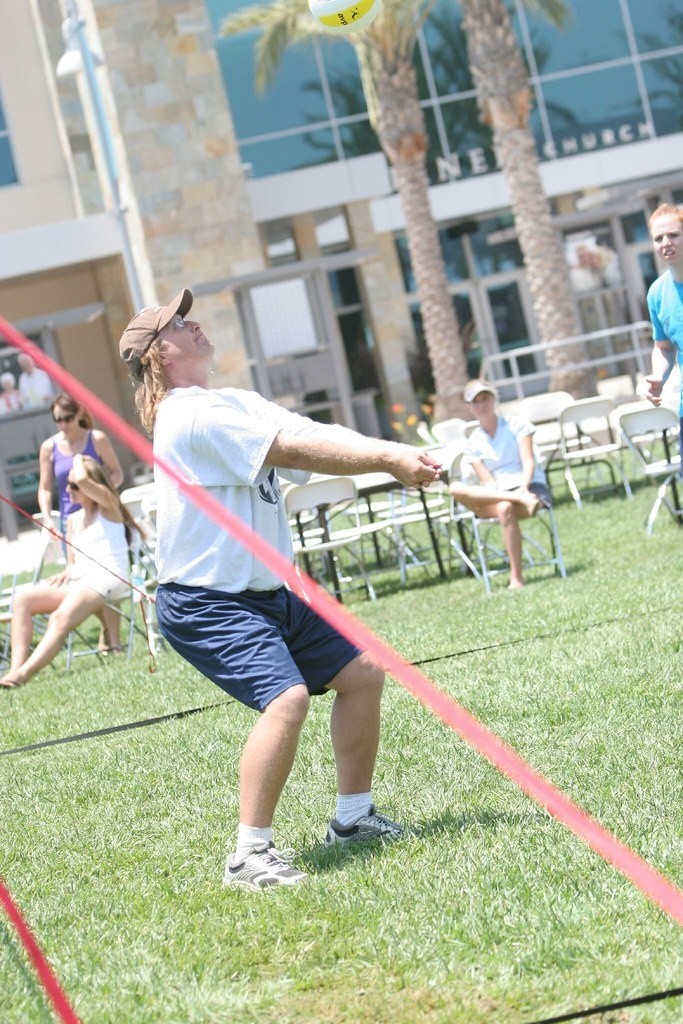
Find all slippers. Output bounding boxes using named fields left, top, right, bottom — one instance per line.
left=1, top=679, right=19, bottom=692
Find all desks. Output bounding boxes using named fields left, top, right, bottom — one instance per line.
left=295, top=470, right=474, bottom=604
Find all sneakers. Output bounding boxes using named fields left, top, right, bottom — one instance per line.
left=324, top=804, right=406, bottom=849
left=223, top=839, right=310, bottom=897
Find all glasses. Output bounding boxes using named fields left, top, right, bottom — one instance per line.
left=158, top=317, right=187, bottom=347
left=65, top=477, right=80, bottom=492
left=54, top=412, right=79, bottom=424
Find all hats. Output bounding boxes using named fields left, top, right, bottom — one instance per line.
left=463, top=383, right=496, bottom=404
left=119, top=288, right=193, bottom=378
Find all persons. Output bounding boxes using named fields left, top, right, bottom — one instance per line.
left=0, top=395, right=135, bottom=692
left=119, top=289, right=443, bottom=891
left=0, top=352, right=53, bottom=414
left=641, top=202, right=683, bottom=467
left=451, top=377, right=548, bottom=590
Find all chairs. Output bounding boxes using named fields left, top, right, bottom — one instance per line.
left=0, top=482, right=169, bottom=676
left=277, top=376, right=682, bottom=595
